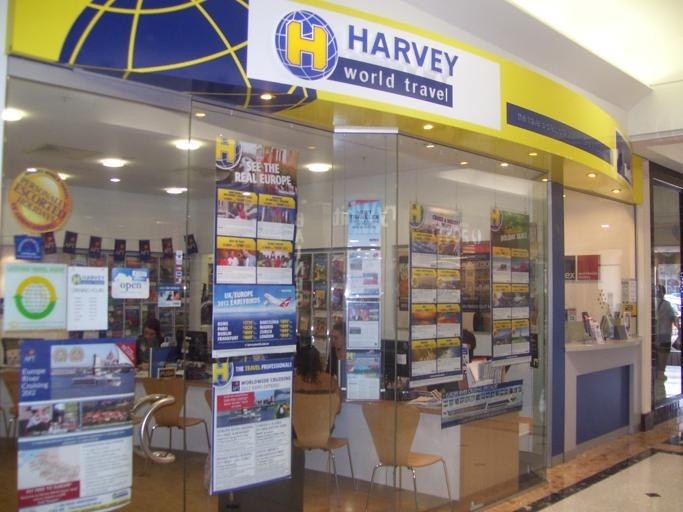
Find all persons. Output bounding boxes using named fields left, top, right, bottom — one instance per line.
left=217, top=203, right=291, bottom=269
left=657, top=284, right=682, bottom=381
left=325, top=320, right=346, bottom=382
left=133, top=318, right=174, bottom=370
left=293, top=345, right=342, bottom=440
left=20, top=404, right=60, bottom=437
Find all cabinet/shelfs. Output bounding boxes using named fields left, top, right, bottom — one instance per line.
left=70, top=248, right=189, bottom=339
left=294, top=245, right=530, bottom=379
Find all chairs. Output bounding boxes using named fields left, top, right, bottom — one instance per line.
left=177, top=332, right=206, bottom=362
left=131, top=406, right=152, bottom=453
left=360, top=398, right=449, bottom=509
left=286, top=388, right=357, bottom=509
left=201, top=387, right=214, bottom=416
left=138, top=376, right=210, bottom=469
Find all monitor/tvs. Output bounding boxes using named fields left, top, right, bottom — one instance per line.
left=176, top=329, right=208, bottom=363
left=381, top=339, right=410, bottom=377
